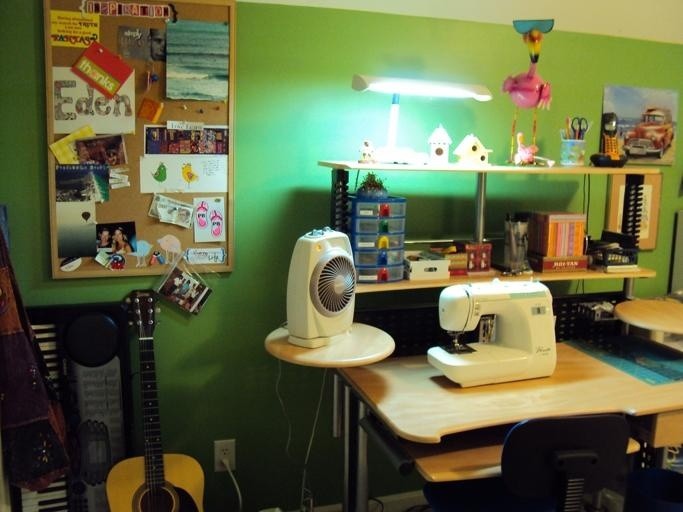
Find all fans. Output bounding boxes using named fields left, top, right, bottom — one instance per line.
left=287, top=227, right=361, bottom=347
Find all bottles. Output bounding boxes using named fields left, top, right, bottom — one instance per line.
left=504, top=211, right=530, bottom=274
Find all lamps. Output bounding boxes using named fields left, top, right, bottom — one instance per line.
left=353, top=73, right=494, bottom=168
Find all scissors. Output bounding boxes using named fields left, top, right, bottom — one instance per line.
left=571, top=117, right=588, bottom=132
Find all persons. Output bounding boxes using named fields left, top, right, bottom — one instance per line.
left=177, top=210, right=191, bottom=224
left=165, top=274, right=204, bottom=310
left=96, top=228, right=128, bottom=254
left=148, top=27, right=167, bottom=63
left=111, top=226, right=132, bottom=254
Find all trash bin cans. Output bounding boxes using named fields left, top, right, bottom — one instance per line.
left=627, top=469, right=682, bottom=511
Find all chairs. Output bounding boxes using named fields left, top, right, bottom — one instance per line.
left=499, top=414, right=642, bottom=511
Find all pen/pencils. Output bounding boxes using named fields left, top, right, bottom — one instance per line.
left=565, top=114, right=571, bottom=139
left=576, top=128, right=585, bottom=140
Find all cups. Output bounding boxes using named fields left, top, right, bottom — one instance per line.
left=560, top=140, right=585, bottom=166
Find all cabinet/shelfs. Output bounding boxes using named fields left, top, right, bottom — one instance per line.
left=320, top=161, right=663, bottom=303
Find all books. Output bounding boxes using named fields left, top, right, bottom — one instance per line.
left=516, top=211, right=588, bottom=258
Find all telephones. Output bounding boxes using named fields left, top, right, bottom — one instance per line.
left=590, top=113, right=627, bottom=167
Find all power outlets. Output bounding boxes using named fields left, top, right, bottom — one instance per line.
left=214, top=439, right=237, bottom=474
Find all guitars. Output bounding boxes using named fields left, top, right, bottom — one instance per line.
left=107, top=290, right=206, bottom=511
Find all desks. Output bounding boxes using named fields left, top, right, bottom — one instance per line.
left=340, top=306, right=683, bottom=511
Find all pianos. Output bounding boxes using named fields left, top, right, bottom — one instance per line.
left=9, top=303, right=135, bottom=512
